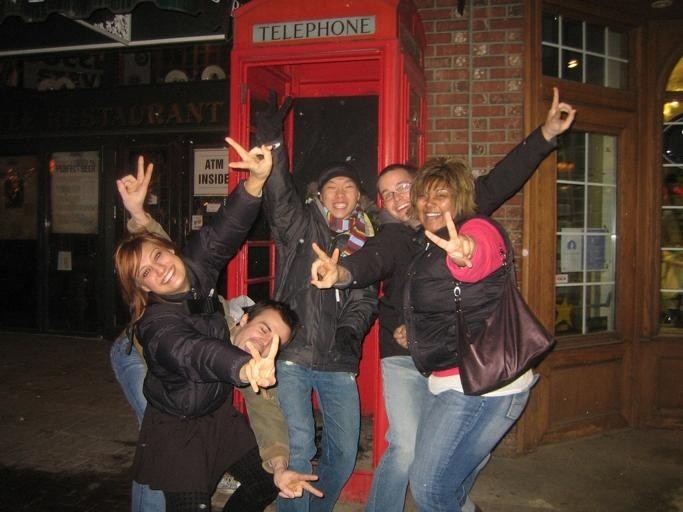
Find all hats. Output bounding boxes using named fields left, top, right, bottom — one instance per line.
left=318, top=162, right=361, bottom=192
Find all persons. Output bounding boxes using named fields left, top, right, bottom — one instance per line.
left=111, top=136, right=282, bottom=511
left=251, top=88, right=382, bottom=510
left=110, top=293, right=325, bottom=512
left=309, top=85, right=579, bottom=512
left=391, top=155, right=557, bottom=511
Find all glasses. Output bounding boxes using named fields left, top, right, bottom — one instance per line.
left=382, top=181, right=413, bottom=201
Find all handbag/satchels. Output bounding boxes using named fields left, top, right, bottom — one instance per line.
left=454, top=259, right=558, bottom=396
